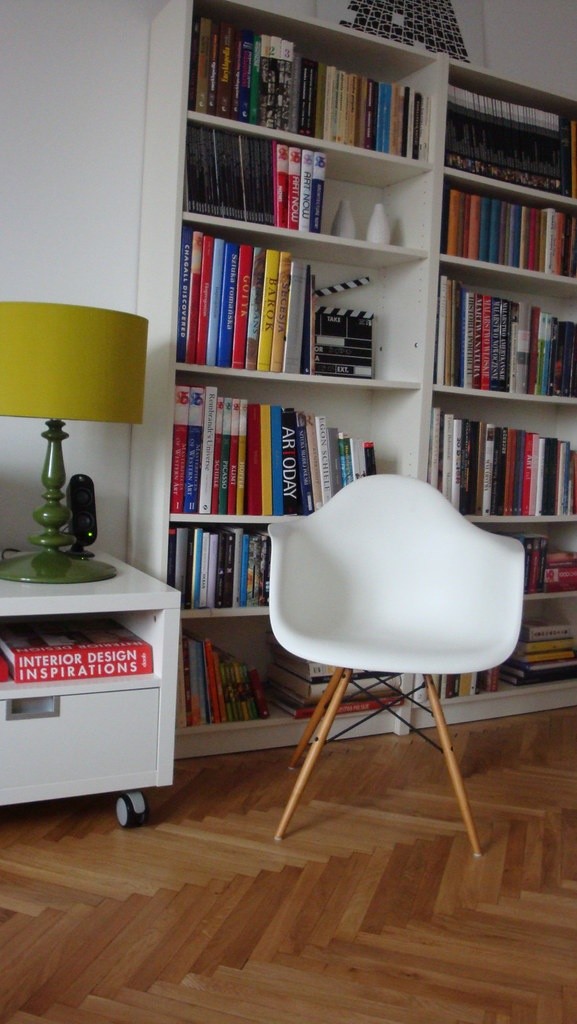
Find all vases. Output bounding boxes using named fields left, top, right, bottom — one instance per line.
left=331, top=200, right=355, bottom=239
left=366, top=203, right=391, bottom=244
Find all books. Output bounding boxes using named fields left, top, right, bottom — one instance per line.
left=444, top=83, right=577, bottom=200
left=175, top=632, right=270, bottom=728
left=167, top=526, right=270, bottom=610
left=176, top=228, right=317, bottom=375
left=187, top=15, right=430, bottom=161
left=0, top=618, right=154, bottom=683
left=170, top=384, right=377, bottom=516
left=427, top=407, right=577, bottom=516
left=440, top=180, right=577, bottom=278
left=498, top=620, right=577, bottom=685
left=412, top=665, right=498, bottom=703
left=433, top=275, right=577, bottom=397
left=491, top=531, right=577, bottom=594
left=269, top=640, right=404, bottom=719
left=183, top=123, right=326, bottom=234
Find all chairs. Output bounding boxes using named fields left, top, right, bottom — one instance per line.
left=268, top=474, right=526, bottom=859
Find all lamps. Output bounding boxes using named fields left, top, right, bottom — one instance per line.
left=0, top=302, right=149, bottom=583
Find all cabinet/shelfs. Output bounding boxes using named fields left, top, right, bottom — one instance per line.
left=130, top=0, right=577, bottom=757
left=0, top=548, right=181, bottom=827
